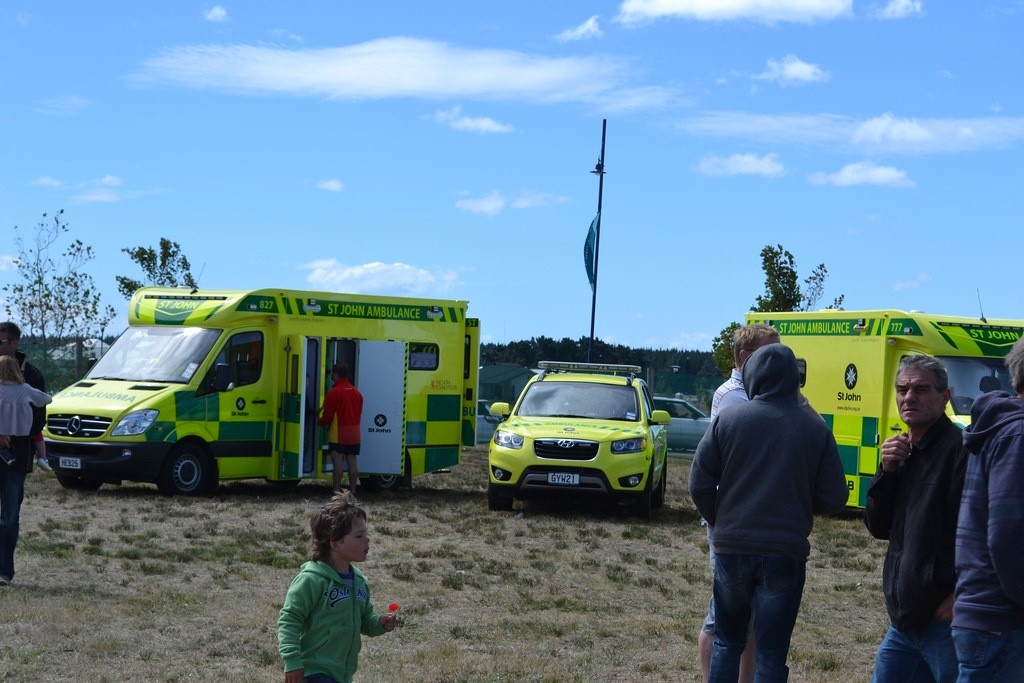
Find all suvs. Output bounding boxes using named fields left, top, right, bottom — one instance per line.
left=486, top=360, right=670, bottom=520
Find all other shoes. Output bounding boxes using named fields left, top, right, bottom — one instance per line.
left=0, top=575, right=13, bottom=584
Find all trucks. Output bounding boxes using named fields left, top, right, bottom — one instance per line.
left=41, top=285, right=483, bottom=499
left=742, top=308, right=1024, bottom=510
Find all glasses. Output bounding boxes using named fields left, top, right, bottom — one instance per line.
left=0, top=339, right=8, bottom=344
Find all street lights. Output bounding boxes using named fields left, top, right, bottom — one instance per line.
left=671, top=363, right=680, bottom=397
left=584, top=118, right=608, bottom=363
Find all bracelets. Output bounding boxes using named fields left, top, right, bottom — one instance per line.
left=798, top=398, right=809, bottom=409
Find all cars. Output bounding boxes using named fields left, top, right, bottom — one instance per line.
left=696, top=383, right=715, bottom=397
left=652, top=396, right=711, bottom=453
left=476, top=399, right=505, bottom=442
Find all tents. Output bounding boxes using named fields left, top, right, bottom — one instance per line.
left=476, top=362, right=538, bottom=444
left=47, top=339, right=111, bottom=362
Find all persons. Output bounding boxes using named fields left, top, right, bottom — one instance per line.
left=953, top=334, right=1024, bottom=683
left=0, top=320, right=48, bottom=585
left=696, top=323, right=828, bottom=682
left=275, top=505, right=398, bottom=683
left=304, top=372, right=314, bottom=469
left=866, top=356, right=971, bottom=683
left=0, top=355, right=55, bottom=469
left=317, top=359, right=365, bottom=503
left=688, top=343, right=853, bottom=683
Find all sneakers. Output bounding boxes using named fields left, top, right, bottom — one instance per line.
left=36, top=458, right=53, bottom=472
